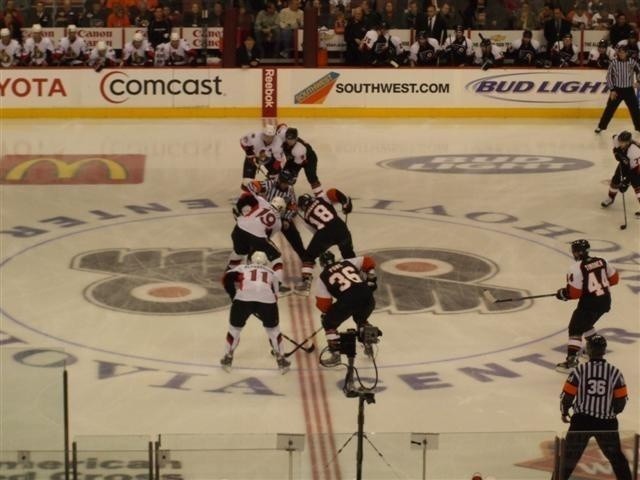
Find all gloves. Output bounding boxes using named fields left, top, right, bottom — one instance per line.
left=342, top=204, right=352, bottom=214
left=247, top=153, right=256, bottom=165
left=365, top=275, right=377, bottom=291
left=556, top=288, right=568, bottom=300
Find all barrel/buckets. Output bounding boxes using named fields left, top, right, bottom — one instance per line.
left=318, top=48, right=328, bottom=65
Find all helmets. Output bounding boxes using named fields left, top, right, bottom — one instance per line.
left=587, top=335, right=607, bottom=355
left=618, top=131, right=631, bottom=141
left=271, top=170, right=313, bottom=211
left=0, top=23, right=179, bottom=51
left=263, top=125, right=298, bottom=139
left=379, top=20, right=532, bottom=46
left=572, top=240, right=590, bottom=261
left=252, top=251, right=268, bottom=265
left=598, top=39, right=607, bottom=47
left=319, top=253, right=335, bottom=266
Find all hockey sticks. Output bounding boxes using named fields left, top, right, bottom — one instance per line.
left=271, top=326, right=323, bottom=358
left=619, top=161, right=627, bottom=230
left=254, top=313, right=315, bottom=353
left=483, top=288, right=556, bottom=303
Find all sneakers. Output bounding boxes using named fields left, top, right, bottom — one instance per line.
left=595, top=127, right=602, bottom=132
left=222, top=353, right=233, bottom=364
left=277, top=356, right=291, bottom=366
left=272, top=281, right=310, bottom=293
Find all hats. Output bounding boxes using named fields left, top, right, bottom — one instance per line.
left=563, top=33, right=572, bottom=38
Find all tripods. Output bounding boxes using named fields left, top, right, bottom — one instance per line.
left=315, top=397, right=402, bottom=480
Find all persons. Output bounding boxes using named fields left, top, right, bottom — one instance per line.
left=594, top=45, right=640, bottom=133
left=225, top=124, right=355, bottom=295
left=0, top=1, right=226, bottom=67
left=233, top=1, right=639, bottom=69
left=219, top=251, right=292, bottom=365
left=315, top=249, right=377, bottom=367
left=600, top=129, right=640, bottom=216
left=558, top=239, right=619, bottom=369
left=560, top=333, right=631, bottom=479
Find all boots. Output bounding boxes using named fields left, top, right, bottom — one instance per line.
left=635, top=197, right=640, bottom=216
left=601, top=190, right=617, bottom=206
left=557, top=349, right=579, bottom=368
left=321, top=348, right=341, bottom=364
left=365, top=344, right=373, bottom=355
